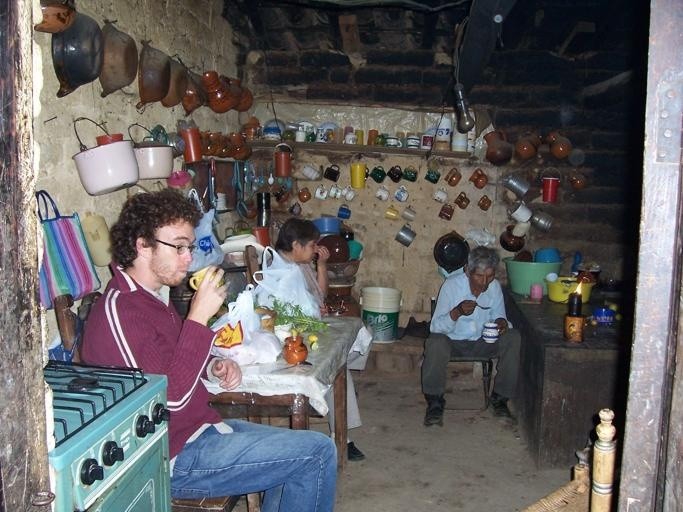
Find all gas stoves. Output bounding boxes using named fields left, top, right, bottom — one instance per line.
left=42, top=359, right=170, bottom=512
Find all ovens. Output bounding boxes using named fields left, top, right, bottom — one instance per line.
left=84, top=431, right=171, bottom=512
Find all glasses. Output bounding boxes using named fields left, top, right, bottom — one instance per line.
left=154, top=239, right=198, bottom=255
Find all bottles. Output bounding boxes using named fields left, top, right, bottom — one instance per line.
left=482, top=128, right=573, bottom=166
left=203, top=72, right=253, bottom=113
left=284, top=336, right=306, bottom=364
left=430, top=297, right=436, bottom=319
left=567, top=174, right=586, bottom=190
left=240, top=119, right=476, bottom=154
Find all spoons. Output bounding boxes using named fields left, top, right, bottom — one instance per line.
left=477, top=305, right=491, bottom=309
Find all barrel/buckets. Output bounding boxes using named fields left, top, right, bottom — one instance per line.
left=72, top=117, right=138, bottom=196
left=357, top=285, right=403, bottom=344
left=129, top=124, right=174, bottom=180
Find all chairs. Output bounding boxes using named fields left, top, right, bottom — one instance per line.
left=429, top=294, right=494, bottom=413
left=57, top=290, right=260, bottom=511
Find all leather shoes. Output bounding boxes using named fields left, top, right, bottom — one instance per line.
left=489, top=390, right=512, bottom=421
left=347, top=442, right=365, bottom=461
left=424, top=395, right=446, bottom=428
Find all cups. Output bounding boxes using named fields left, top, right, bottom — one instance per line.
left=80, top=216, right=115, bottom=267
left=299, top=161, right=492, bottom=248
left=215, top=192, right=227, bottom=211
left=175, top=127, right=200, bottom=165
left=501, top=174, right=560, bottom=238
left=256, top=191, right=271, bottom=227
left=188, top=266, right=227, bottom=292
left=530, top=284, right=544, bottom=301
left=481, top=323, right=500, bottom=344
left=545, top=273, right=558, bottom=283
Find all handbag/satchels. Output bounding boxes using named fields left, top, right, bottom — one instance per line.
left=186, top=189, right=224, bottom=271
left=36, top=190, right=101, bottom=309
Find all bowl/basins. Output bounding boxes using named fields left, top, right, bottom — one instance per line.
left=543, top=276, right=597, bottom=304
left=534, top=247, right=561, bottom=263
left=311, top=233, right=364, bottom=285
left=502, top=256, right=565, bottom=297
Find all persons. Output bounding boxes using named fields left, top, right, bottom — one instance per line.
left=420, top=245, right=523, bottom=428
left=80, top=189, right=339, bottom=512
left=257, top=218, right=374, bottom=463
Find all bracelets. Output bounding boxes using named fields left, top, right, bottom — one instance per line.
left=453, top=307, right=462, bottom=317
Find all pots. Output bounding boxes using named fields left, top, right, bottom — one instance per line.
left=51, top=11, right=205, bottom=117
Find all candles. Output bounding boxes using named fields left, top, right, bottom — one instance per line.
left=565, top=280, right=586, bottom=317
